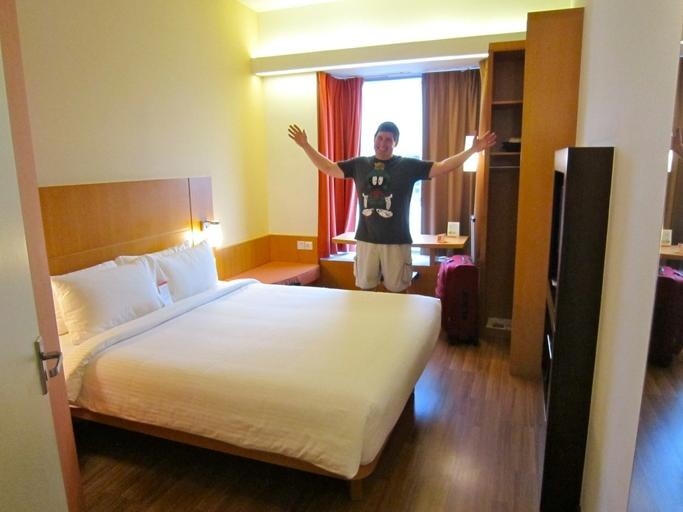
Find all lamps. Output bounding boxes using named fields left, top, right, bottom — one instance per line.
left=200, top=218, right=223, bottom=246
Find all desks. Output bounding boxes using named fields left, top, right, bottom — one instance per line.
left=661, top=241, right=683, bottom=267
left=319, top=230, right=469, bottom=297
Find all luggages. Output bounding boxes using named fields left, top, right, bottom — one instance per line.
left=649, top=263, right=683, bottom=370
left=435, top=215, right=477, bottom=346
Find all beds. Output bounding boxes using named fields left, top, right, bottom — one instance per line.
left=37, top=175, right=443, bottom=500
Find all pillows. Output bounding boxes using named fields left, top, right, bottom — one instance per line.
left=160, top=242, right=219, bottom=303
left=49, top=259, right=116, bottom=334
left=49, top=254, right=164, bottom=346
left=116, top=239, right=196, bottom=286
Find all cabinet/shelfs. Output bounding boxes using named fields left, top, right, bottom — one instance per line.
left=473, top=39, right=525, bottom=317
left=536, top=145, right=614, bottom=512
left=509, top=9, right=582, bottom=382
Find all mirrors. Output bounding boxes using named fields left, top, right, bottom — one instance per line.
left=624, top=33, right=683, bottom=512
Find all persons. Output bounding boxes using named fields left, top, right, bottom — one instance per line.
left=285, top=116, right=498, bottom=296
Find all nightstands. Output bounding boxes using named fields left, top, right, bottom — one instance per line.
left=224, top=261, right=321, bottom=286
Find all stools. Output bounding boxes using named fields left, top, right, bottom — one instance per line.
left=379, top=269, right=419, bottom=294
